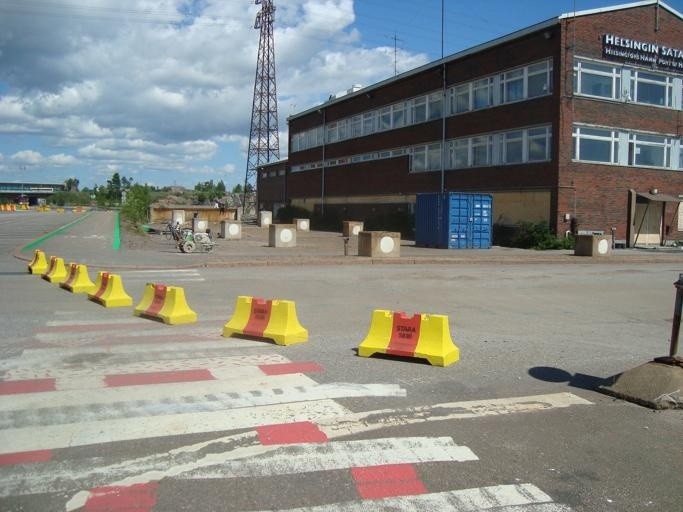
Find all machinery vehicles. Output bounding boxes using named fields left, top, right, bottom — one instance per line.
left=166, top=223, right=216, bottom=254
